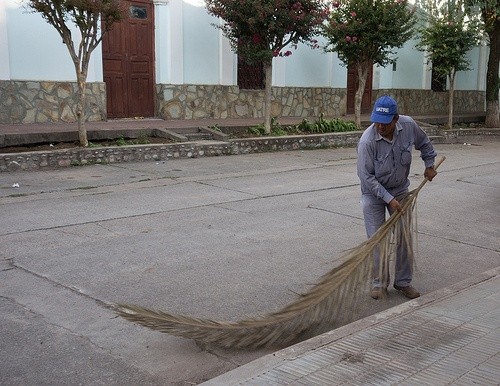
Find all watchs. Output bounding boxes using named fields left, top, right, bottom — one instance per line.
left=429, top=163, right=435, bottom=169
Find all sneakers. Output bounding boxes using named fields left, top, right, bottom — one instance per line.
left=372, top=287, right=387, bottom=299
left=393, top=283, right=420, bottom=299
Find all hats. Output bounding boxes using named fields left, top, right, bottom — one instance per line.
left=370, top=95, right=398, bottom=124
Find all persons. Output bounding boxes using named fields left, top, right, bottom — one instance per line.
left=357, top=95, right=438, bottom=299
